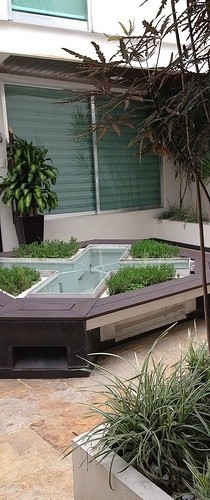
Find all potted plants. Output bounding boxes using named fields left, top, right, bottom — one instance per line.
left=0, top=134, right=59, bottom=246
